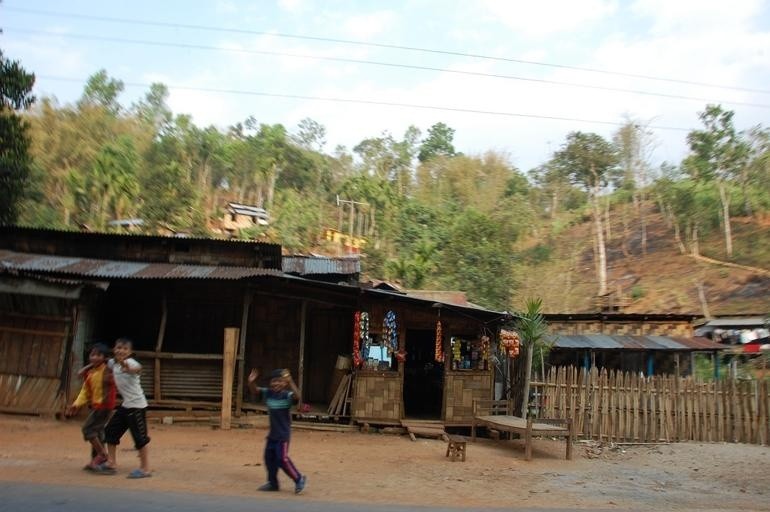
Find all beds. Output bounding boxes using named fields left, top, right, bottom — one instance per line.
left=470, top=395, right=573, bottom=462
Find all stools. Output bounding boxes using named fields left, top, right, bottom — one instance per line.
left=446, top=434, right=466, bottom=461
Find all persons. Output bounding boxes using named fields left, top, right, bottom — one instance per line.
left=65, top=343, right=118, bottom=470
left=77, top=338, right=154, bottom=480
left=246, top=364, right=307, bottom=495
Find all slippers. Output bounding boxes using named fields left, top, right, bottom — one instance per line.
left=127, top=468, right=153, bottom=478
left=294, top=473, right=306, bottom=493
left=256, top=481, right=279, bottom=491
left=84, top=455, right=119, bottom=474
